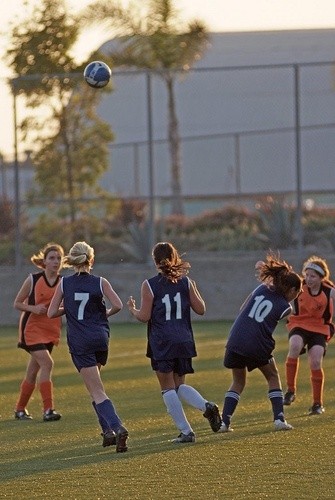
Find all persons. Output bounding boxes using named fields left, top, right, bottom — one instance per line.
left=13, top=241, right=66, bottom=422
left=217, top=252, right=303, bottom=432
left=126, top=242, right=221, bottom=443
left=283, top=256, right=335, bottom=414
left=46, top=241, right=130, bottom=453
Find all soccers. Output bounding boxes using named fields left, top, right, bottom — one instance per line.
left=84, top=61, right=112, bottom=89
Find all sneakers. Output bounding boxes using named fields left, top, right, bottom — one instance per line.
left=168, top=432, right=195, bottom=443
left=203, top=402, right=222, bottom=433
left=100, top=430, right=116, bottom=447
left=308, top=403, right=325, bottom=415
left=219, top=426, right=234, bottom=432
left=283, top=392, right=296, bottom=405
left=274, top=419, right=293, bottom=430
left=43, top=409, right=61, bottom=422
left=15, top=410, right=33, bottom=420
left=116, top=427, right=129, bottom=453
